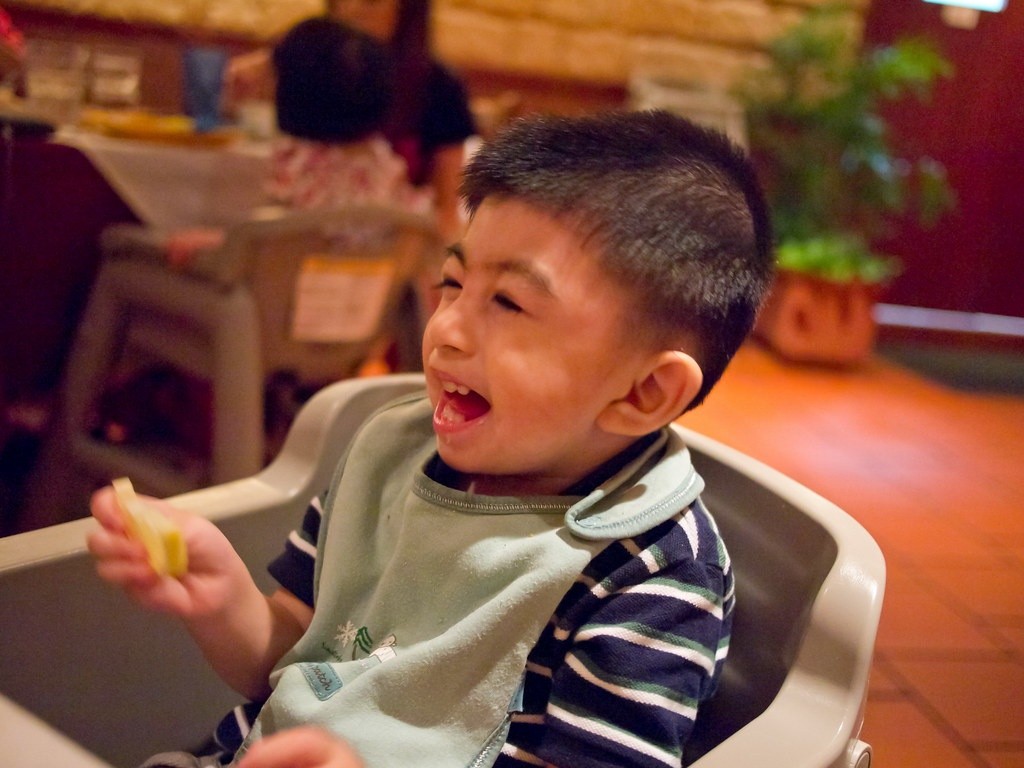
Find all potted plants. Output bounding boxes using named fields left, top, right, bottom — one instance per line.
left=772, top=236, right=894, bottom=365
left=738, top=0, right=952, bottom=350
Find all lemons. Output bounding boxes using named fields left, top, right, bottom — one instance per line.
left=113, top=476, right=187, bottom=576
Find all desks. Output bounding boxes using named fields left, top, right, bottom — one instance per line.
left=0, top=120, right=282, bottom=399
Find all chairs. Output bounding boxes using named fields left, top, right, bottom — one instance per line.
left=0, top=370, right=889, bottom=768
left=25, top=197, right=454, bottom=528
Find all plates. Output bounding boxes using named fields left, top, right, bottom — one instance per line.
left=81, top=106, right=194, bottom=138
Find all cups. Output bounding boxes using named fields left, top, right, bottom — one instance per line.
left=90, top=45, right=145, bottom=104
left=24, top=39, right=88, bottom=109
left=240, top=101, right=278, bottom=142
left=184, top=50, right=223, bottom=131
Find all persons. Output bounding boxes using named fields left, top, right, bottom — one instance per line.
left=172, top=50, right=427, bottom=266
left=0, top=10, right=300, bottom=502
left=273, top=18, right=491, bottom=240
left=0, top=108, right=776, bottom=768
